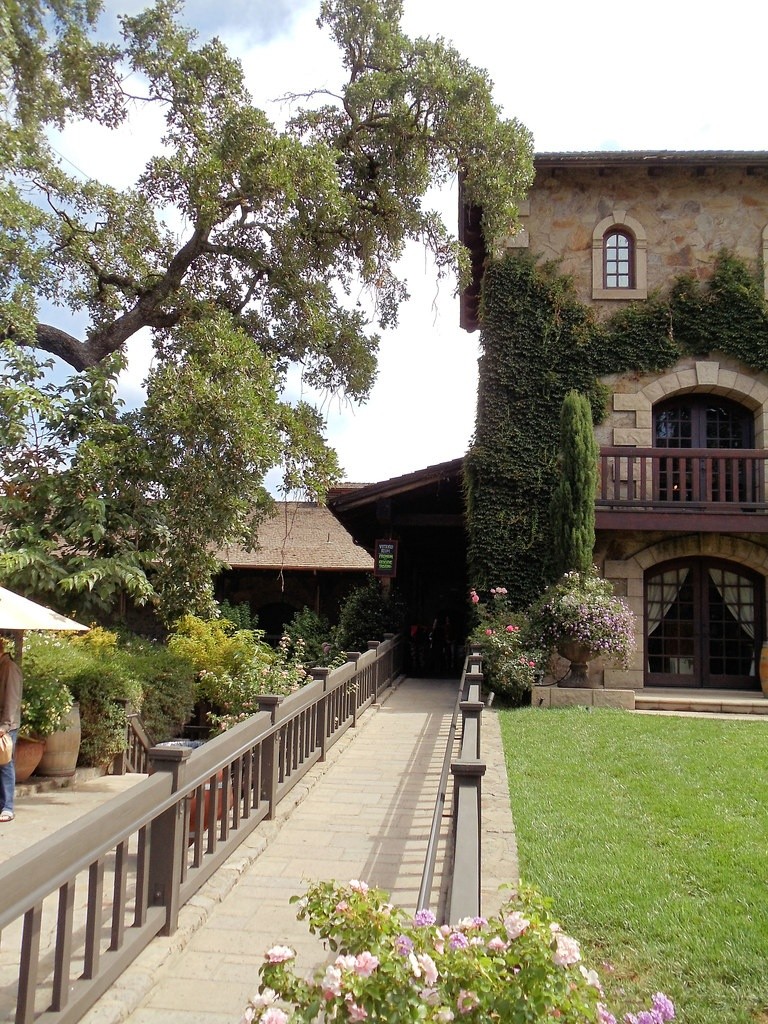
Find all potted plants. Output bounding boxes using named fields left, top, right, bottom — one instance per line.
left=13, top=732, right=46, bottom=784
left=553, top=637, right=607, bottom=690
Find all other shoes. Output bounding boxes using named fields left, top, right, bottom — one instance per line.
left=1, top=809, right=17, bottom=822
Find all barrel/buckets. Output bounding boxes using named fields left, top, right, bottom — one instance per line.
left=153, top=739, right=235, bottom=848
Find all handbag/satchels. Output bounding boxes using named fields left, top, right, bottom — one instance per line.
left=0, top=732, right=13, bottom=766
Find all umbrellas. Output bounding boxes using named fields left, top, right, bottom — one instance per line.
left=0, top=586, right=92, bottom=631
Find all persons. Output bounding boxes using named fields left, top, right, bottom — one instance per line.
left=406, top=609, right=452, bottom=674
left=0, top=638, right=25, bottom=822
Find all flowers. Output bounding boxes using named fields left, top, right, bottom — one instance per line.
left=541, top=572, right=635, bottom=671
left=19, top=665, right=78, bottom=738
